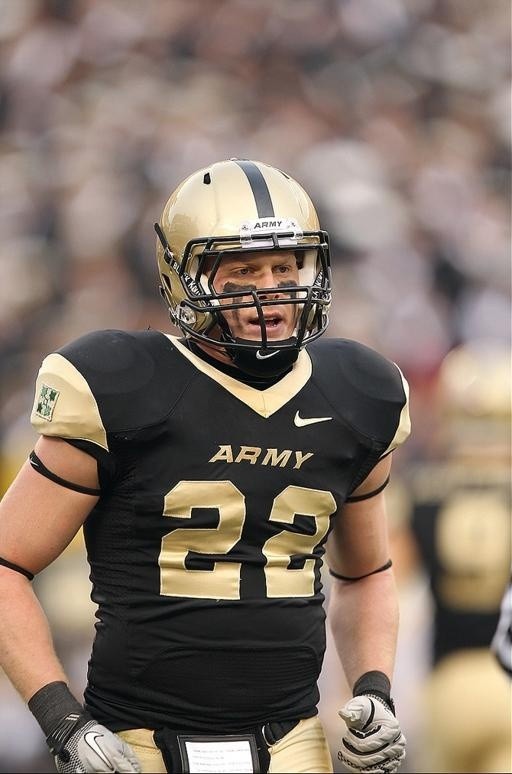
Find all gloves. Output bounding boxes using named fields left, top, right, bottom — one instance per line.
left=337, top=670, right=406, bottom=774
left=28, top=680, right=140, bottom=774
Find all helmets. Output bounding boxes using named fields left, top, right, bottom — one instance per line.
left=156, top=158, right=321, bottom=340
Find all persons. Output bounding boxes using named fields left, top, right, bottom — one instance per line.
left=386, top=337, right=512, bottom=774
left=0, top=158, right=412, bottom=774
